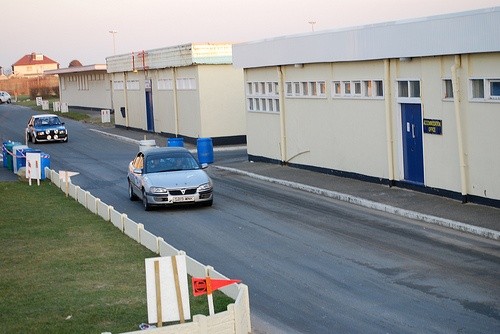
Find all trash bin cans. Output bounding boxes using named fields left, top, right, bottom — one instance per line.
left=2, top=141, right=51, bottom=181
left=167, top=138, right=184, bottom=147
left=196, top=137, right=215, bottom=164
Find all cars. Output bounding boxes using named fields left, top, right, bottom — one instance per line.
left=26, top=114, right=68, bottom=143
left=127, top=147, right=214, bottom=211
left=0, top=91, right=11, bottom=104
